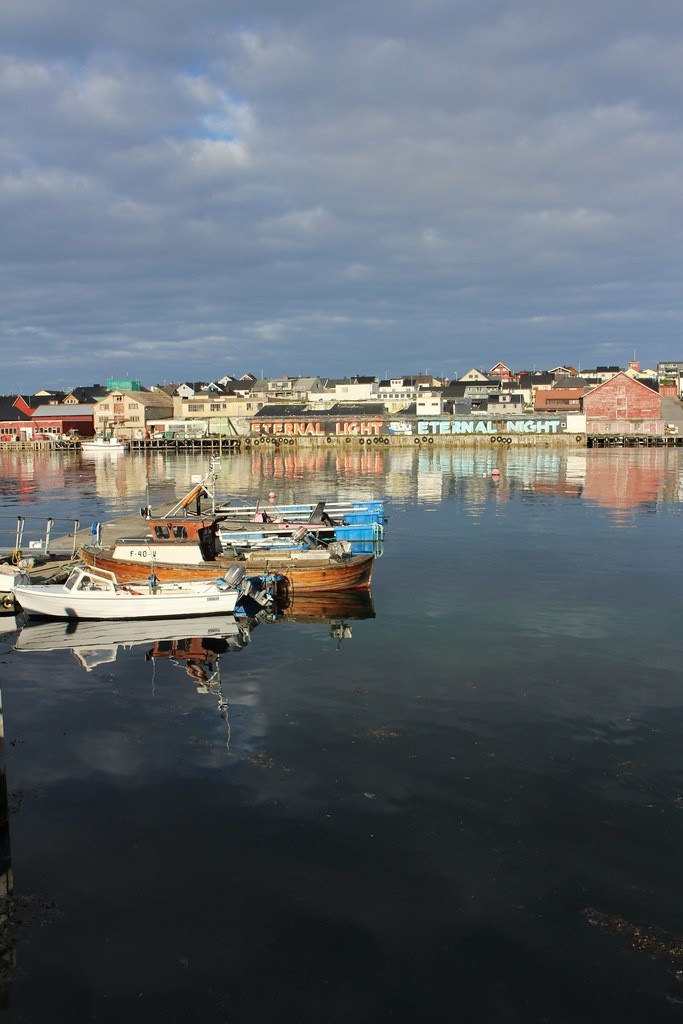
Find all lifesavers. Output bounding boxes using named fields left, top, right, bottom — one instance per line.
left=593, top=436, right=597, bottom=442
left=327, top=437, right=331, bottom=443
left=245, top=438, right=250, bottom=443
left=260, top=437, right=265, bottom=443
left=284, top=439, right=288, bottom=444
left=272, top=438, right=276, bottom=444
left=605, top=437, right=609, bottom=442
left=625, top=437, right=629, bottom=443
left=278, top=438, right=283, bottom=442
left=614, top=437, right=618, bottom=442
left=275, top=442, right=279, bottom=446
left=491, top=436, right=512, bottom=444
left=254, top=440, right=259, bottom=445
left=266, top=438, right=270, bottom=442
left=289, top=440, right=294, bottom=444
left=576, top=436, right=581, bottom=441
left=635, top=437, right=639, bottom=442
left=414, top=437, right=433, bottom=443
left=346, top=438, right=350, bottom=442
left=359, top=437, right=389, bottom=444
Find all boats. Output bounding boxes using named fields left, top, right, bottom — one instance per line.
left=10, top=617, right=249, bottom=671
left=81, top=437, right=128, bottom=451
left=0, top=453, right=390, bottom=622
left=144, top=586, right=376, bottom=750
